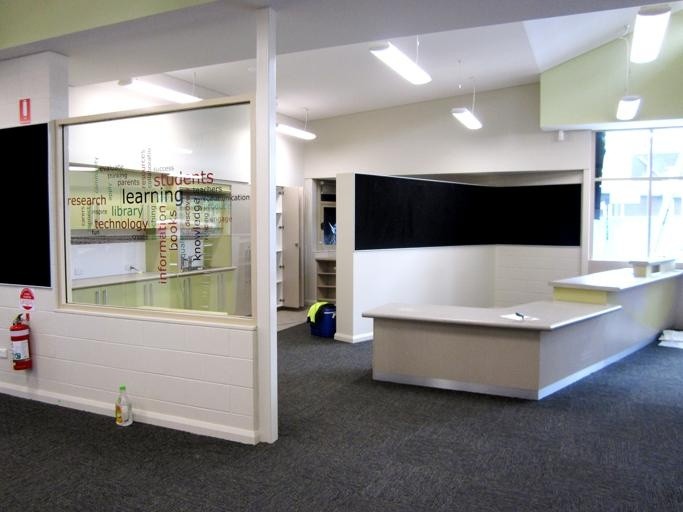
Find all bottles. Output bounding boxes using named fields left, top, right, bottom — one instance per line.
left=114, top=383, right=132, bottom=427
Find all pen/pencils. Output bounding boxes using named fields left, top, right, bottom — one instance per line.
left=515, top=311, right=524, bottom=318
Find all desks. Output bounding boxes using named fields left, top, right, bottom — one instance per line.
left=360, top=270, right=683, bottom=402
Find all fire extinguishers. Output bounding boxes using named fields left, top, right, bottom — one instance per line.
left=9, top=314, right=33, bottom=370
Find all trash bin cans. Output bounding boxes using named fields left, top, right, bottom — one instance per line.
left=311, top=305, right=336, bottom=337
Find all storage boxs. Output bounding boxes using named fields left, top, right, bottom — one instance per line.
left=306, top=304, right=335, bottom=339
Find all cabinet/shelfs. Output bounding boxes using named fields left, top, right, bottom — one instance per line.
left=209, top=269, right=235, bottom=315
left=124, top=278, right=164, bottom=308
left=274, top=185, right=303, bottom=309
left=72, top=283, right=121, bottom=309
left=313, top=256, right=335, bottom=302
left=165, top=274, right=208, bottom=313
